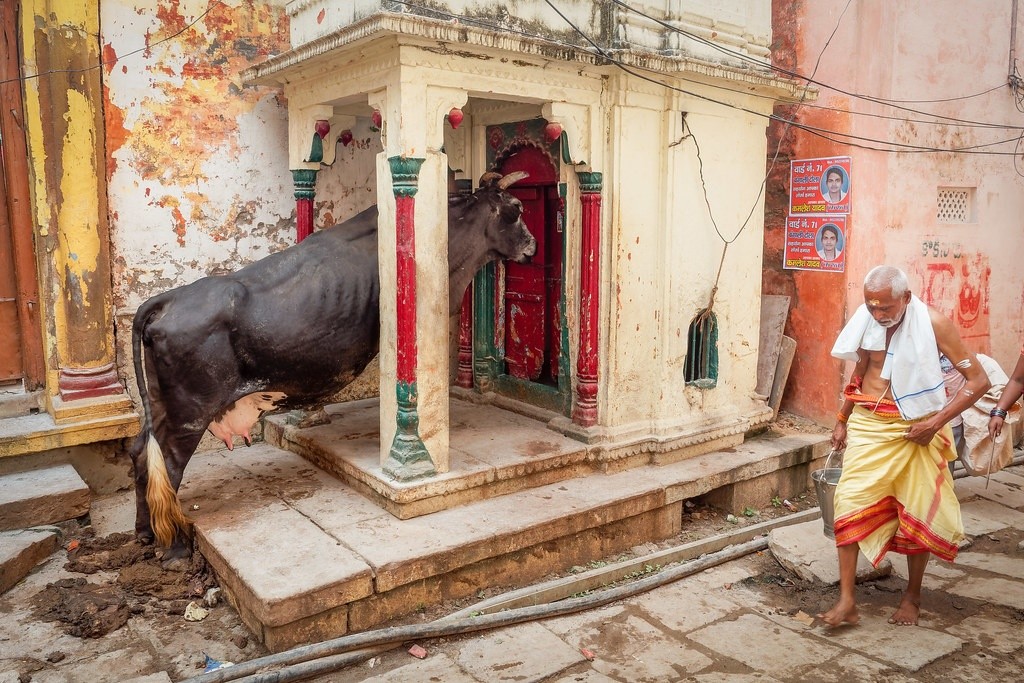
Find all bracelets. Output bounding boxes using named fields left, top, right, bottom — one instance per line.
left=837, top=411, right=848, bottom=424
left=990, top=407, right=1007, bottom=420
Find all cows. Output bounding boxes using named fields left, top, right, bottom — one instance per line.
left=130, top=169, right=538, bottom=573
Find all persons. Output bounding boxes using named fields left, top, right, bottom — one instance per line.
left=816, top=266, right=991, bottom=626
left=822, top=168, right=846, bottom=203
left=817, top=226, right=841, bottom=260
left=988, top=343, right=1024, bottom=442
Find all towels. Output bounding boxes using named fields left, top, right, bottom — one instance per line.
left=831, top=293, right=947, bottom=421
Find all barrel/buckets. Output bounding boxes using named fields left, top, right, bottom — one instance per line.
left=810, top=447, right=843, bottom=540
